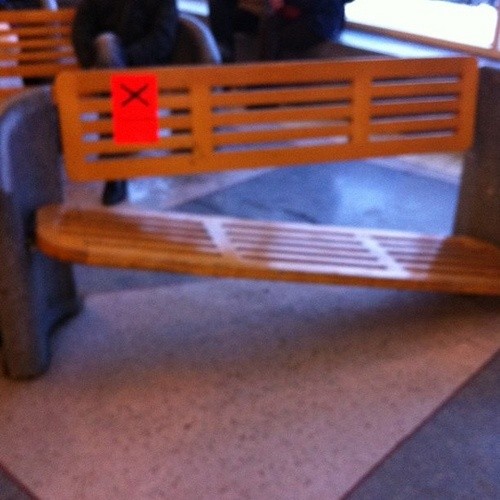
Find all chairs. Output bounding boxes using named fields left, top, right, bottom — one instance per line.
left=0, top=8, right=76, bottom=116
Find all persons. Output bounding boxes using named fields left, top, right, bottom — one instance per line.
left=69, top=0, right=196, bottom=204
left=205, top=0, right=354, bottom=110
